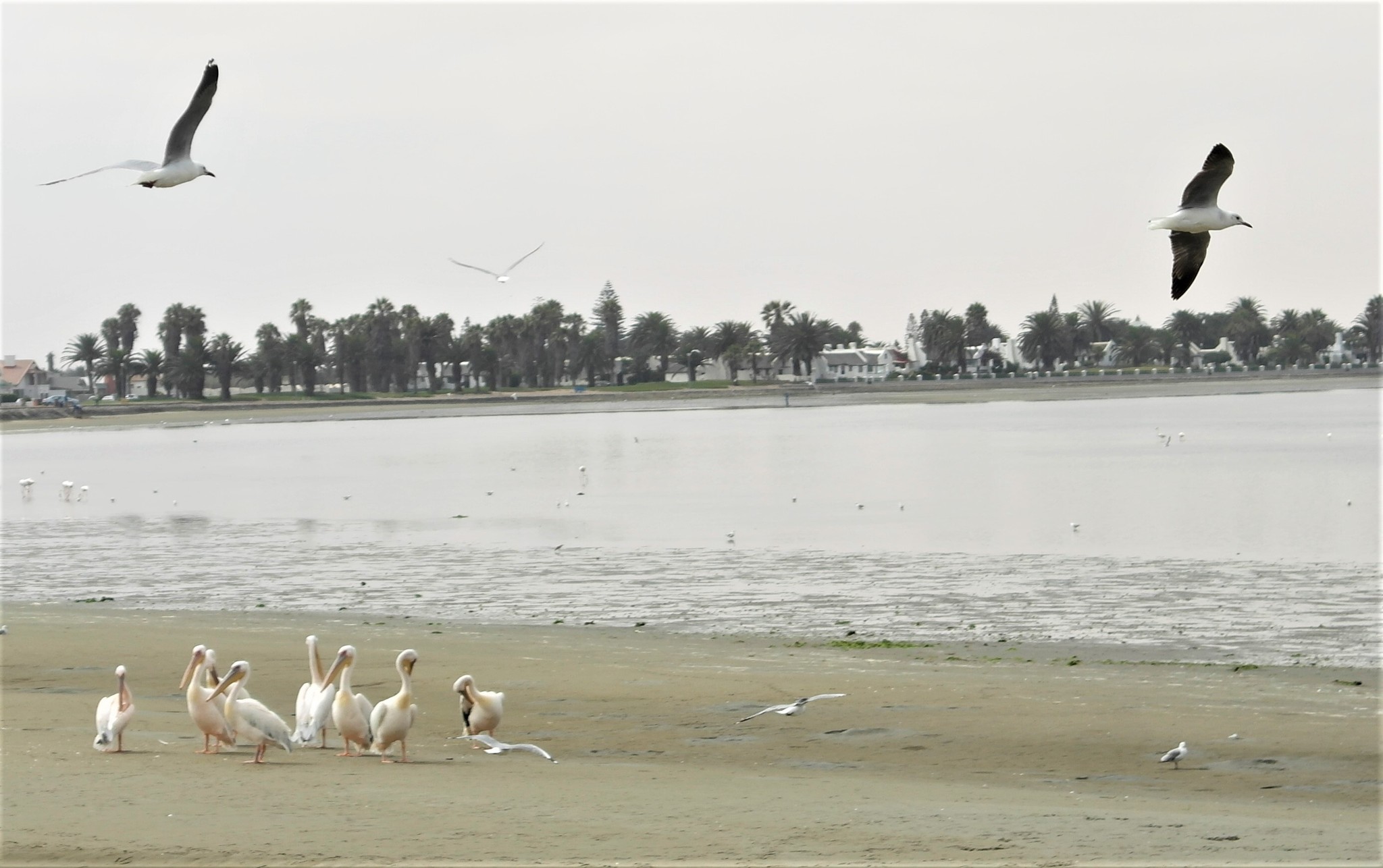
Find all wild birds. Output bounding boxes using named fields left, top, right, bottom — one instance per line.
left=555, top=544, right=563, bottom=552
left=555, top=466, right=586, bottom=508
left=179, top=644, right=293, bottom=763
left=94, top=665, right=134, bottom=752
left=735, top=694, right=847, bottom=725
left=20, top=478, right=35, bottom=500
left=450, top=241, right=546, bottom=283
left=0, top=625, right=8, bottom=635
left=447, top=735, right=559, bottom=764
left=1154, top=427, right=1184, bottom=446
left=60, top=481, right=89, bottom=503
left=1147, top=143, right=1253, bottom=300
left=319, top=645, right=419, bottom=764
left=1158, top=742, right=1187, bottom=770
left=1070, top=522, right=1080, bottom=531
left=37, top=57, right=219, bottom=189
left=727, top=530, right=735, bottom=539
left=1327, top=432, right=1331, bottom=437
left=289, top=635, right=336, bottom=749
left=453, top=675, right=504, bottom=749
